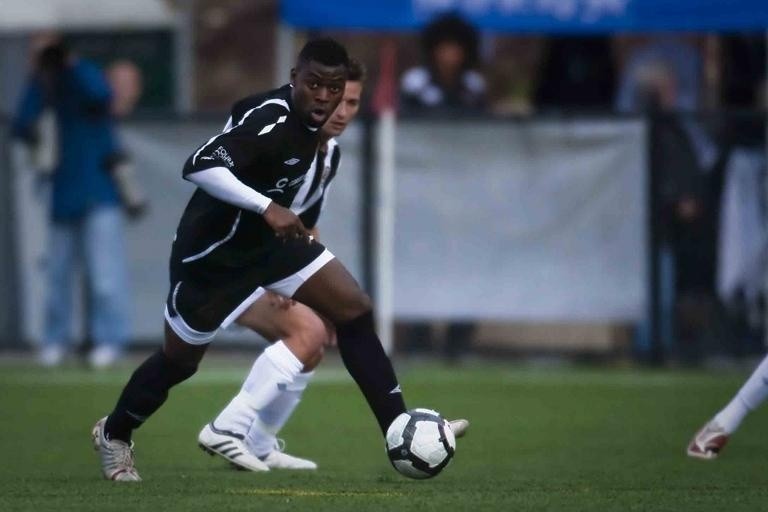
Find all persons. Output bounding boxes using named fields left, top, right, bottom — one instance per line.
left=90, top=33, right=469, bottom=483
left=195, top=58, right=363, bottom=473
left=687, top=355, right=766, bottom=460
left=396, top=6, right=497, bottom=358
left=7, top=38, right=151, bottom=373
left=525, top=19, right=615, bottom=110
left=101, top=51, right=146, bottom=122
left=611, top=49, right=720, bottom=355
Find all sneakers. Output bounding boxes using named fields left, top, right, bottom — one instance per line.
left=686, top=422, right=728, bottom=458
left=91, top=416, right=142, bottom=482
left=198, top=423, right=318, bottom=472
left=449, top=418, right=469, bottom=438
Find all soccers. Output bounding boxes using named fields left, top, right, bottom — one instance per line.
left=385, top=408, right=456, bottom=480
